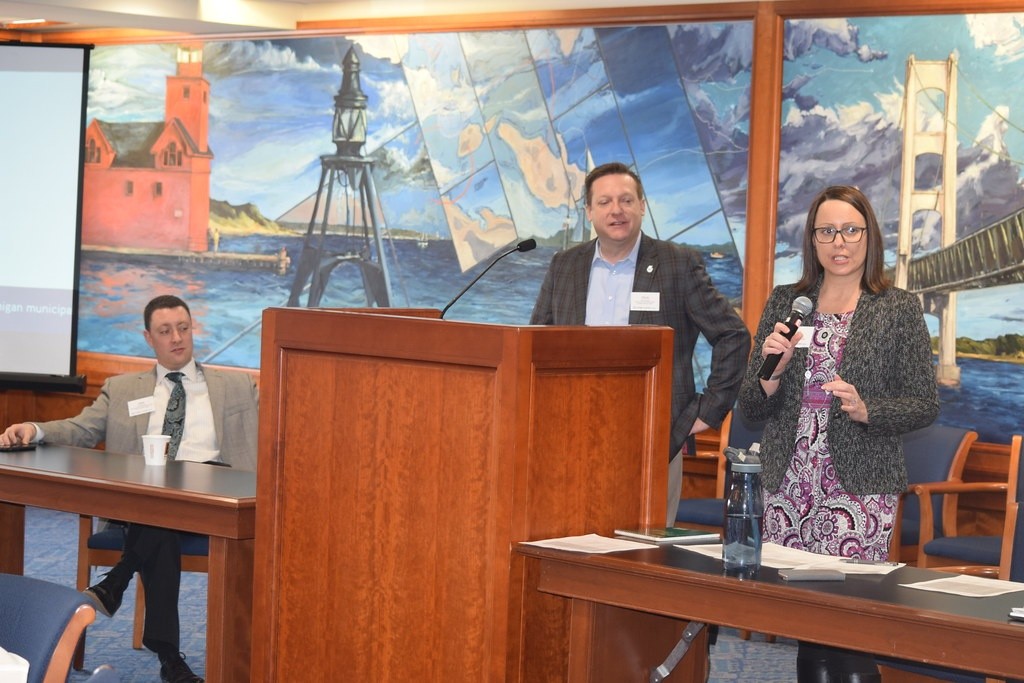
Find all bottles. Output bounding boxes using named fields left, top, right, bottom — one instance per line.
left=721, top=440, right=766, bottom=573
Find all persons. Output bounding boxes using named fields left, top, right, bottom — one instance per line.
left=0, top=294, right=258, bottom=683
left=528, top=164, right=750, bottom=682
left=739, top=185, right=940, bottom=683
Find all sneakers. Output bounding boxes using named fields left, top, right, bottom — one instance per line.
left=85, top=579, right=124, bottom=617
left=157, top=655, right=201, bottom=681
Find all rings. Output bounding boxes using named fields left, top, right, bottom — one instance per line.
left=851, top=398, right=858, bottom=404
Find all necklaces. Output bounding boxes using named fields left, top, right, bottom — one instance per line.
left=806, top=296, right=859, bottom=411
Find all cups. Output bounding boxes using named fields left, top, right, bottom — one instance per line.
left=142, top=434, right=172, bottom=467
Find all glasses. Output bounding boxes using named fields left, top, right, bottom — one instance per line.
left=815, top=226, right=867, bottom=245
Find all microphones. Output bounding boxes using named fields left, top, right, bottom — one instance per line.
left=757, top=295, right=813, bottom=382
left=439, top=238, right=536, bottom=319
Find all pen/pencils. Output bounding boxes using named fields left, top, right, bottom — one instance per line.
left=840, top=558, right=899, bottom=567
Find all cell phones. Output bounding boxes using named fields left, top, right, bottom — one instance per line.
left=0, top=444, right=37, bottom=453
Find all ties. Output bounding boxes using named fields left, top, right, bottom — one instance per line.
left=160, top=372, right=185, bottom=461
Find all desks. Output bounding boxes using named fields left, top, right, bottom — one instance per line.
left=2, top=304, right=1022, bottom=681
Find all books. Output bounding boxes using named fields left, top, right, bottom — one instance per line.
left=613, top=526, right=720, bottom=541
